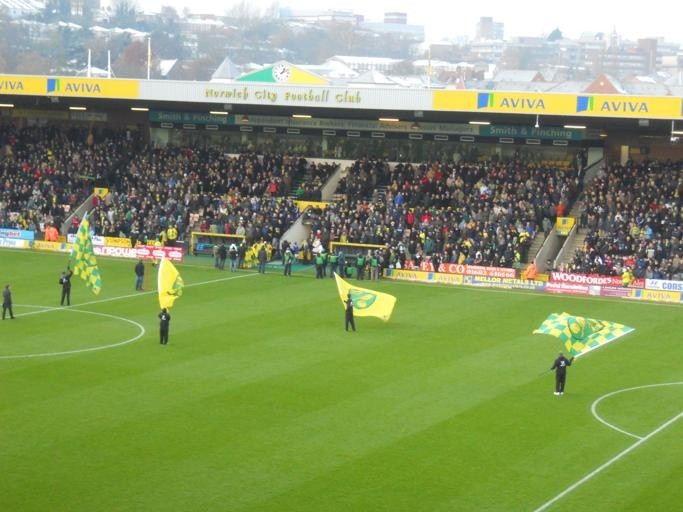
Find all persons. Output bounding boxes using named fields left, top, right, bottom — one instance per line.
left=58, top=266, right=72, bottom=305
left=342, top=293, right=355, bottom=333
left=1, top=284, right=15, bottom=320
left=157, top=308, right=169, bottom=344
left=549, top=350, right=574, bottom=395
left=0, top=114, right=683, bottom=290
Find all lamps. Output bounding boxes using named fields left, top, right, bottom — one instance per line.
left=241, top=106, right=249, bottom=122
left=600, top=119, right=609, bottom=137
left=411, top=118, right=422, bottom=130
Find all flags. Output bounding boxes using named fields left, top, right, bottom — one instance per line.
left=334, top=272, right=397, bottom=322
left=426, top=49, right=432, bottom=76
left=532, top=312, right=635, bottom=355
left=147, top=50, right=153, bottom=68
left=157, top=256, right=183, bottom=313
left=67, top=213, right=102, bottom=295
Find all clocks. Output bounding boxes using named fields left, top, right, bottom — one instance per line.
left=272, top=60, right=292, bottom=83
